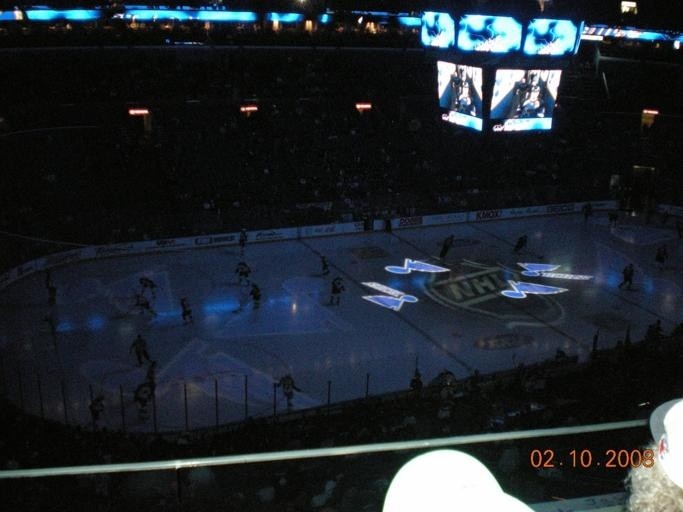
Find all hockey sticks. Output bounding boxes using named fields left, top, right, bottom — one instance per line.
left=118, top=304, right=136, bottom=320
left=232, top=298, right=249, bottom=313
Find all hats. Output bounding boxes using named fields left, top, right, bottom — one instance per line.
left=649, top=396, right=683, bottom=487
left=383, top=449, right=533, bottom=512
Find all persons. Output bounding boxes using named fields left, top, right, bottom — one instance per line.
left=0, top=12, right=682, bottom=512
